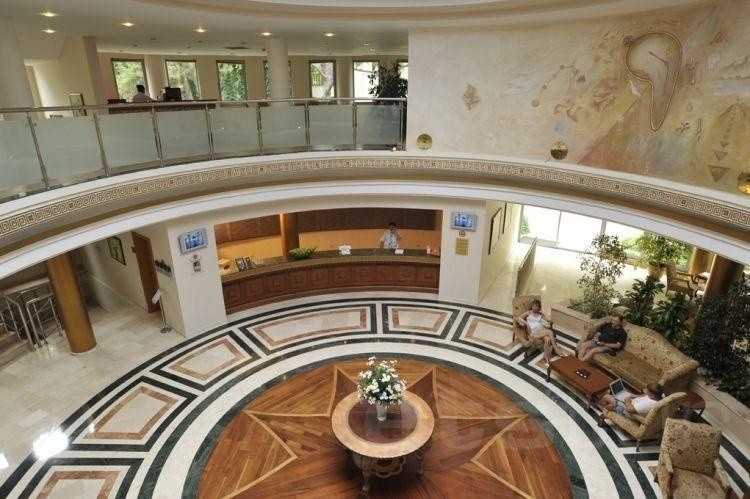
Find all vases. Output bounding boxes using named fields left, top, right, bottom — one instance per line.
left=375, top=400, right=388, bottom=422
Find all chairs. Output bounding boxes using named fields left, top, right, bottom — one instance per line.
left=512, top=295, right=554, bottom=359
left=598, top=392, right=689, bottom=452
left=654, top=417, right=732, bottom=499
left=665, top=260, right=698, bottom=302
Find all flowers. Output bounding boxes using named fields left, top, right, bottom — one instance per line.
left=357, top=356, right=407, bottom=409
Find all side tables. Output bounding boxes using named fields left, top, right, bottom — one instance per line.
left=671, top=386, right=705, bottom=423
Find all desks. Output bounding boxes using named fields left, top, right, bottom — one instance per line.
left=332, top=390, right=435, bottom=492
left=694, top=271, right=710, bottom=281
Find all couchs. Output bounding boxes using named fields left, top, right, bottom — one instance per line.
left=576, top=315, right=700, bottom=396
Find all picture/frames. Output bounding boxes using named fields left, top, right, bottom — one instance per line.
left=488, top=207, right=502, bottom=255
left=68, top=93, right=87, bottom=117
left=503, top=202, right=513, bottom=234
left=235, top=257, right=247, bottom=272
left=107, top=236, right=126, bottom=266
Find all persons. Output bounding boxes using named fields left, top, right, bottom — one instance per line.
left=517, top=301, right=568, bottom=364
left=132, top=84, right=156, bottom=101
left=378, top=221, right=401, bottom=249
left=602, top=381, right=665, bottom=422
left=577, top=313, right=627, bottom=363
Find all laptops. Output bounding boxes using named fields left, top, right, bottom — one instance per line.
left=609, top=378, right=632, bottom=403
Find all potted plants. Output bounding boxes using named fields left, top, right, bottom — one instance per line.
left=633, top=231, right=687, bottom=281
